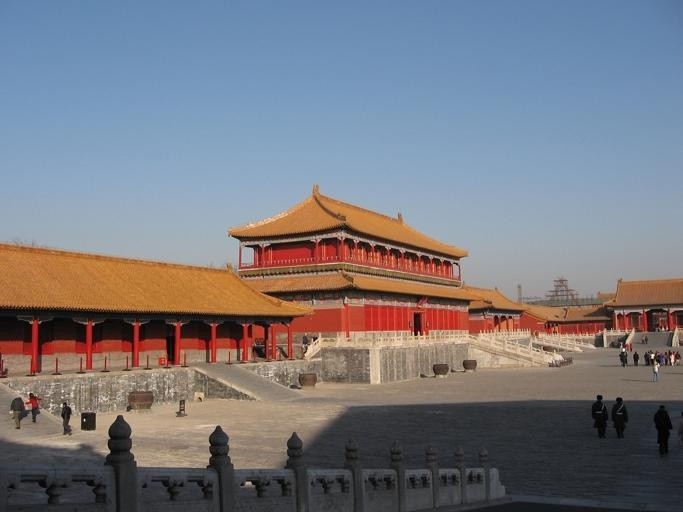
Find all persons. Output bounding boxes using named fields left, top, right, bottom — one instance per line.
left=618, top=342, right=681, bottom=367
left=678, top=411, right=683, bottom=442
left=25, top=392, right=41, bottom=423
left=60, top=401, right=71, bottom=436
left=300, top=332, right=308, bottom=353
left=611, top=396, right=628, bottom=438
left=652, top=361, right=660, bottom=382
left=8, top=395, right=24, bottom=428
left=645, top=336, right=648, bottom=344
left=591, top=394, right=608, bottom=438
left=641, top=336, right=644, bottom=344
left=652, top=405, right=673, bottom=457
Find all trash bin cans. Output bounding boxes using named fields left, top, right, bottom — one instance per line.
left=619, top=342, right=623, bottom=350
left=81, top=412, right=96, bottom=430
